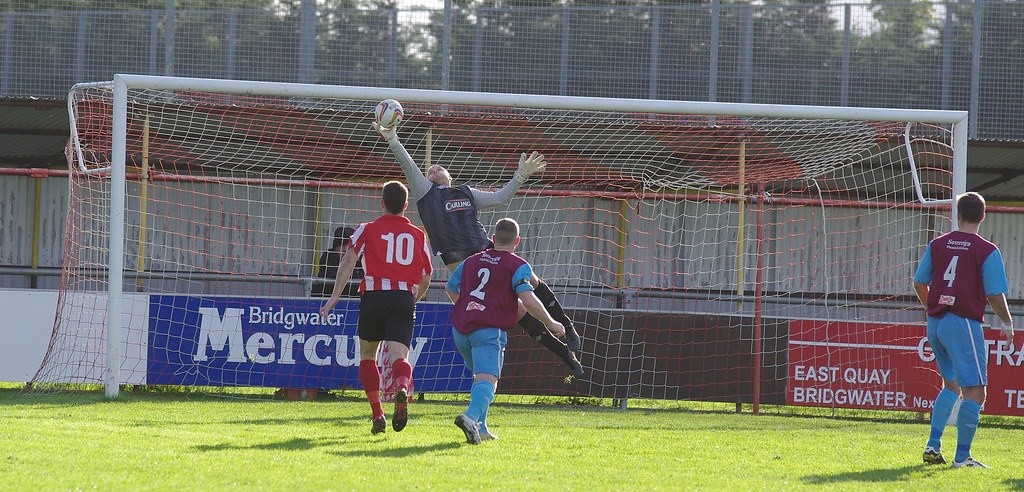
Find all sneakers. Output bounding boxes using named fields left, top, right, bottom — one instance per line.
left=454, top=414, right=483, bottom=445
left=923, top=446, right=947, bottom=465
left=480, top=429, right=500, bottom=441
left=563, top=323, right=581, bottom=350
left=371, top=416, right=386, bottom=433
left=564, top=348, right=583, bottom=376
left=951, top=457, right=988, bottom=468
left=392, top=390, right=408, bottom=432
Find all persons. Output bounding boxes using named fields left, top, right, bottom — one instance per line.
left=913, top=192, right=1014, bottom=469
left=319, top=181, right=432, bottom=434
left=446, top=217, right=565, bottom=445
left=313, top=228, right=364, bottom=298
left=372, top=116, right=583, bottom=377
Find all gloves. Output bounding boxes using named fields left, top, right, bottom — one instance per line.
left=516, top=151, right=547, bottom=182
left=372, top=120, right=397, bottom=142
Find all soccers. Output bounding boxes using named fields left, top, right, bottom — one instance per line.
left=375, top=99, right=404, bottom=128
